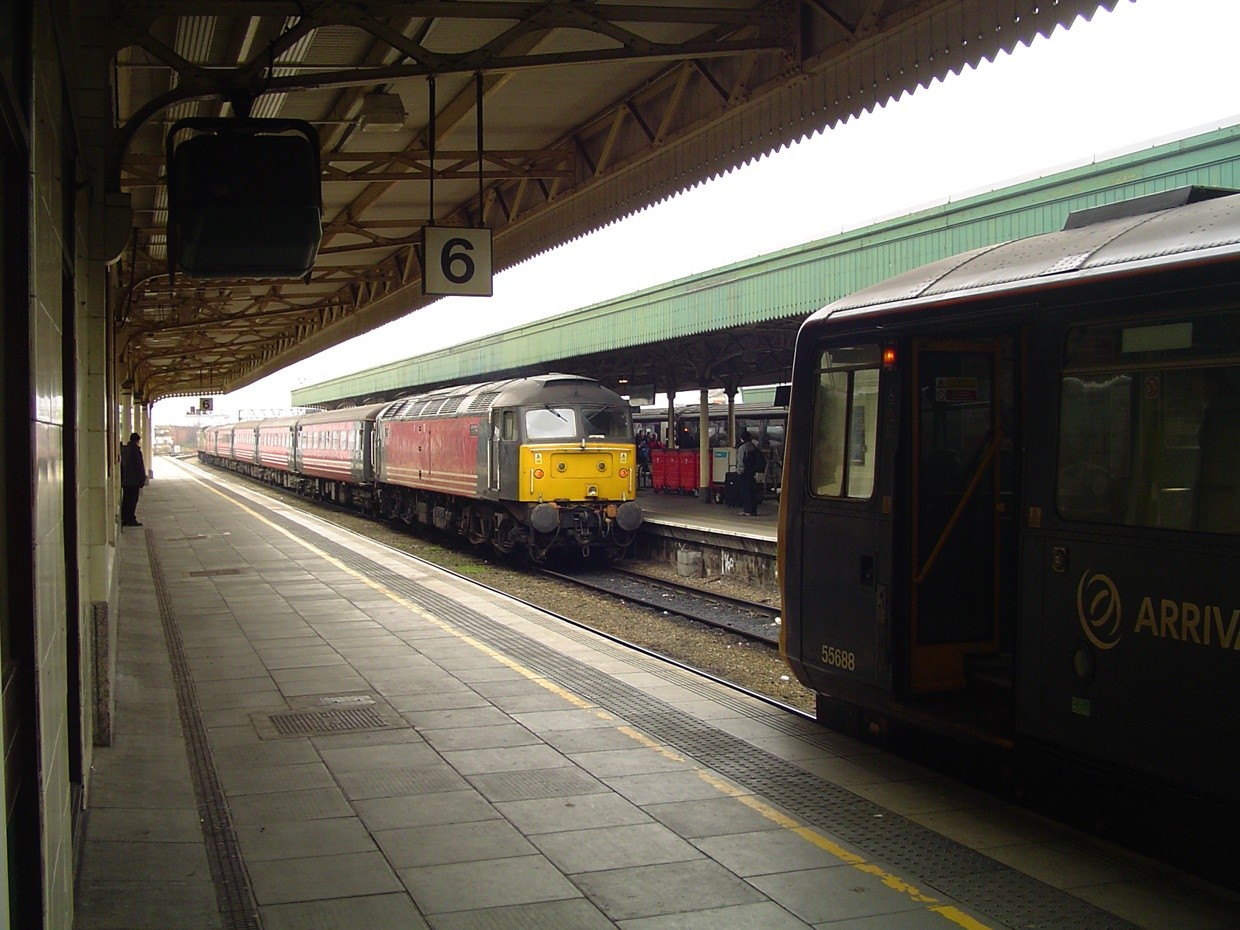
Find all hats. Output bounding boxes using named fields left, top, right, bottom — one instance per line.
left=130, top=433, right=140, bottom=441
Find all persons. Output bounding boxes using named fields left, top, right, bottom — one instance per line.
left=121, top=432, right=146, bottom=526
left=737, top=431, right=757, bottom=516
left=639, top=440, right=649, bottom=474
left=636, top=429, right=665, bottom=463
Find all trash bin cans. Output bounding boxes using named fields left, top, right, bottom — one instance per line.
left=692, top=448, right=715, bottom=499
left=663, top=449, right=680, bottom=495
left=651, top=449, right=673, bottom=493
left=678, top=449, right=697, bottom=496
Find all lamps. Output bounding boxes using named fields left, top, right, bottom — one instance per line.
left=311, top=90, right=411, bottom=133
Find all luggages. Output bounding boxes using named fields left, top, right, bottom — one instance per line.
left=724, top=464, right=743, bottom=509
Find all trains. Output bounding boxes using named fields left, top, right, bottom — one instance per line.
left=630, top=400, right=788, bottom=495
left=195, top=373, right=644, bottom=571
left=777, top=185, right=1240, bottom=859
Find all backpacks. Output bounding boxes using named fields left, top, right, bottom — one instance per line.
left=742, top=442, right=767, bottom=473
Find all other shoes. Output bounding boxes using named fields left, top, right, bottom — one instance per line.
left=124, top=520, right=142, bottom=526
left=738, top=512, right=751, bottom=516
